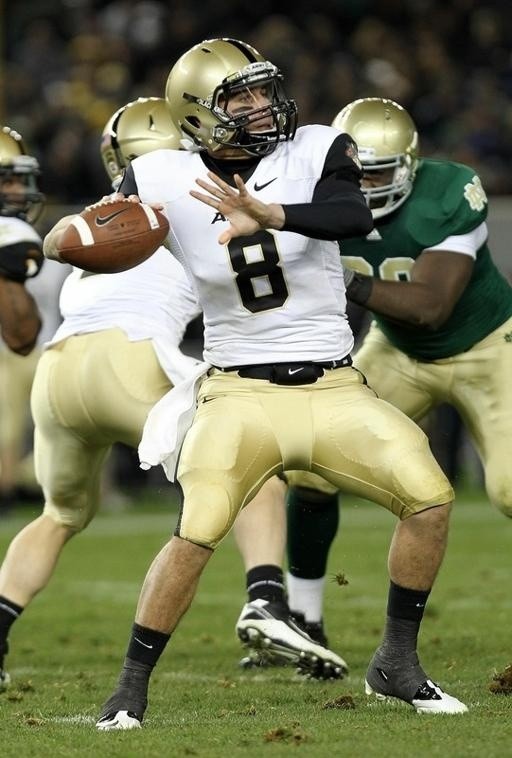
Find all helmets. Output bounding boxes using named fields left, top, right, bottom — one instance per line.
left=332, top=98, right=421, bottom=222
left=99, top=96, right=187, bottom=191
left=167, top=36, right=299, bottom=158
left=1, top=126, right=46, bottom=225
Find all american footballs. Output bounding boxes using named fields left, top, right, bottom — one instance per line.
left=59, top=203, right=171, bottom=275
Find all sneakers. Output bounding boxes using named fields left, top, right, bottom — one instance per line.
left=364, top=646, right=468, bottom=713
left=235, top=597, right=348, bottom=680
left=96, top=684, right=146, bottom=731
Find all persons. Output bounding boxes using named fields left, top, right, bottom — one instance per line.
left=1, top=123, right=45, bottom=356
left=42, top=35, right=472, bottom=734
left=238, top=91, right=510, bottom=673
left=2, top=3, right=512, bottom=206
left=3, top=95, right=354, bottom=685
left=0, top=223, right=73, bottom=493
